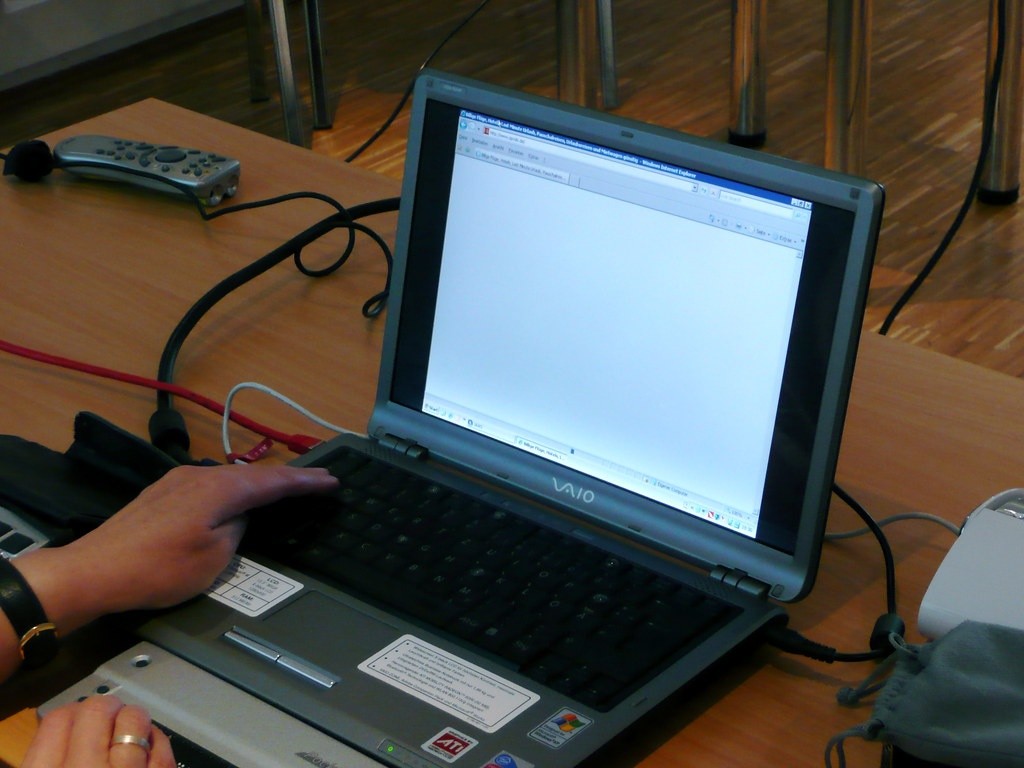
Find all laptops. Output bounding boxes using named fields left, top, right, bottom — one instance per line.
left=25, top=65, right=885, bottom=768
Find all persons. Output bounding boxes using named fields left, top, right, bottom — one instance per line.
left=0, top=465, right=339, bottom=768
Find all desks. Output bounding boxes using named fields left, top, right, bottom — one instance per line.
left=0, top=97, right=1024, bottom=768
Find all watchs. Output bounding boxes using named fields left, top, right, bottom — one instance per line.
left=0, top=554, right=60, bottom=665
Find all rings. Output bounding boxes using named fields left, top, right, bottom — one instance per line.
left=109, top=735, right=151, bottom=753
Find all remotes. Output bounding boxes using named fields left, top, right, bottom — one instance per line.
left=54, top=135, right=242, bottom=205
